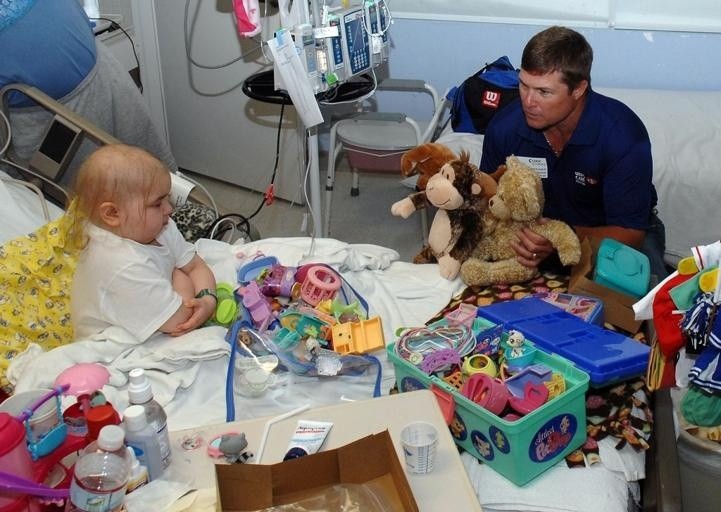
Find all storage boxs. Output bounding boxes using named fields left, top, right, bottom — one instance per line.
left=573, top=236, right=661, bottom=331
left=211, top=426, right=420, bottom=511
left=384, top=293, right=651, bottom=488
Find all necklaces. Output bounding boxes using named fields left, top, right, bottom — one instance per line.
left=538, top=128, right=563, bottom=157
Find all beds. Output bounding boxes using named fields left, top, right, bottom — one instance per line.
left=0, top=81, right=683, bottom=512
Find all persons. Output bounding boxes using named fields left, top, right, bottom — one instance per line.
left=1, top=0, right=180, bottom=212
left=480, top=26, right=669, bottom=291
left=62, top=142, right=218, bottom=344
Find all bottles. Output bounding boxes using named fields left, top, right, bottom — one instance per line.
left=121, top=404, right=164, bottom=482
left=65, top=424, right=134, bottom=512
left=126, top=366, right=173, bottom=471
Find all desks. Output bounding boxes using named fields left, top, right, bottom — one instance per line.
left=169, top=390, right=485, bottom=512
left=240, top=67, right=378, bottom=238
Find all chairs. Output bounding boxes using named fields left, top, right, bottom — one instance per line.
left=323, top=77, right=451, bottom=246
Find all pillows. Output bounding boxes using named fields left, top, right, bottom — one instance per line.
left=0, top=192, right=87, bottom=399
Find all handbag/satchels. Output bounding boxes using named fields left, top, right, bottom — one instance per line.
left=445, top=55, right=520, bottom=134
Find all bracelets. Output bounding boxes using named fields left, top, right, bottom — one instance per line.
left=195, top=288, right=218, bottom=301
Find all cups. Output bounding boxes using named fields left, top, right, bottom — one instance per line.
left=400, top=421, right=439, bottom=475
left=1, top=387, right=62, bottom=445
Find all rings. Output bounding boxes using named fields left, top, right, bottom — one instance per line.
left=531, top=250, right=538, bottom=262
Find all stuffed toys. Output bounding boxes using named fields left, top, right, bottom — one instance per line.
left=391, top=147, right=487, bottom=280
left=398, top=143, right=507, bottom=215
left=461, top=153, right=582, bottom=288
left=219, top=431, right=256, bottom=468
